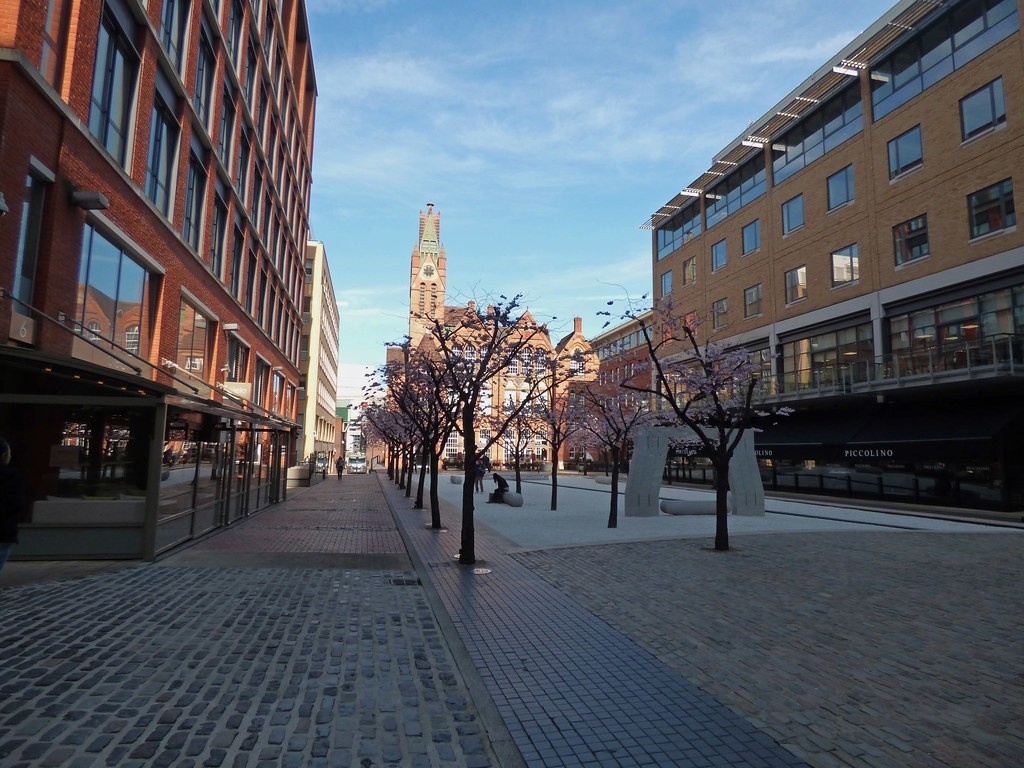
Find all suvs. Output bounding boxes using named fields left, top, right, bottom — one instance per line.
left=315, top=457, right=328, bottom=472
left=442, top=457, right=465, bottom=470
left=348, top=455, right=367, bottom=473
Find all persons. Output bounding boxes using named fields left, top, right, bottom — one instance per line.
left=485, top=472, right=510, bottom=504
left=336, top=456, right=345, bottom=480
left=482, top=455, right=491, bottom=474
left=474, top=458, right=486, bottom=494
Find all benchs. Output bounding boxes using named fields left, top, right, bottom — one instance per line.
left=595, top=476, right=612, bottom=485
left=503, top=490, right=523, bottom=508
left=660, top=500, right=732, bottom=515
left=450, top=475, right=463, bottom=484
